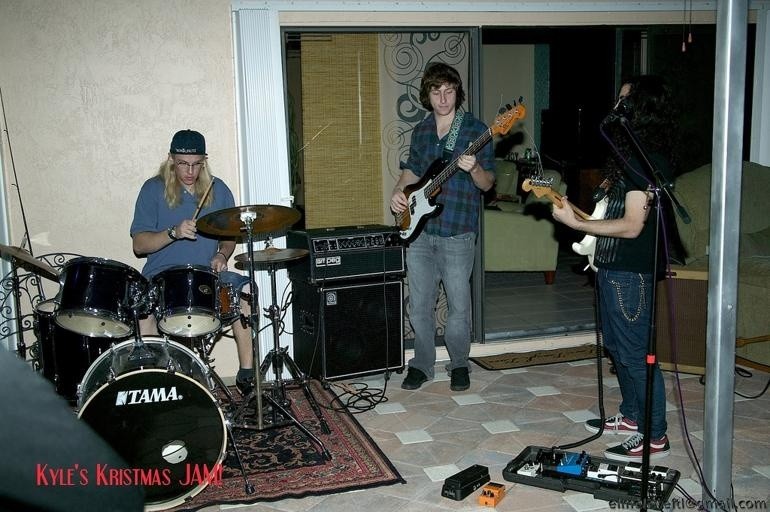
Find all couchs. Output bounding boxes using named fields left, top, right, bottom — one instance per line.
left=671, top=161, right=765, bottom=372
left=481, top=160, right=567, bottom=284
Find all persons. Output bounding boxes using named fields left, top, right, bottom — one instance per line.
left=552, top=76, right=672, bottom=462
left=131, top=130, right=259, bottom=398
left=389, top=64, right=498, bottom=391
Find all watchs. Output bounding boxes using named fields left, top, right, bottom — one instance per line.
left=167, top=226, right=178, bottom=240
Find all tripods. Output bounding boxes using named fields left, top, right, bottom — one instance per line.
left=10, top=264, right=26, bottom=362
left=618, top=116, right=688, bottom=512
left=226, top=218, right=332, bottom=493
left=230, top=264, right=332, bottom=436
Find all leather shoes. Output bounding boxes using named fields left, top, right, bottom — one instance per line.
left=401, top=366, right=429, bottom=390
left=450, top=366, right=470, bottom=391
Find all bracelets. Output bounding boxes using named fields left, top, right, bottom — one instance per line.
left=217, top=252, right=229, bottom=259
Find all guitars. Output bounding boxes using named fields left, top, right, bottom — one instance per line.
left=390, top=95, right=528, bottom=245
left=522, top=175, right=608, bottom=273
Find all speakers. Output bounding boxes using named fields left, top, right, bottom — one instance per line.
left=655, top=267, right=709, bottom=376
left=292, top=278, right=405, bottom=383
left=286, top=224, right=407, bottom=280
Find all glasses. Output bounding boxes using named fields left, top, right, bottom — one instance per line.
left=175, top=159, right=204, bottom=168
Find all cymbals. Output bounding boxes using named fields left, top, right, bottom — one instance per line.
left=234, top=248, right=310, bottom=270
left=0, top=238, right=60, bottom=283
left=197, top=204, right=302, bottom=243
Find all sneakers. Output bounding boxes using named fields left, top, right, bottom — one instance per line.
left=604, top=433, right=671, bottom=462
left=234, top=376, right=270, bottom=411
left=584, top=412, right=639, bottom=435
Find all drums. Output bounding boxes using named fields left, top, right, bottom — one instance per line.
left=150, top=263, right=223, bottom=339
left=75, top=336, right=228, bottom=512
left=33, top=298, right=60, bottom=377
left=54, top=256, right=147, bottom=338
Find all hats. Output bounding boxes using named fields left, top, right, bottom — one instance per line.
left=169, top=129, right=208, bottom=155
